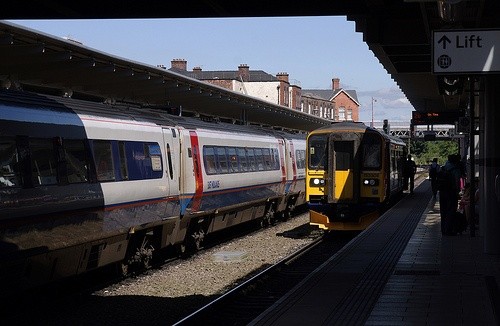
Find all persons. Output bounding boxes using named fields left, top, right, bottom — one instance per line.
left=428, top=157, right=443, bottom=198
left=402, top=154, right=416, bottom=194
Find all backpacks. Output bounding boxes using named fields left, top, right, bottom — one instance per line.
left=429, top=164, right=438, bottom=177
left=436, top=166, right=458, bottom=192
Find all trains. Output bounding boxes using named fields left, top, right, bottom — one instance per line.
left=0, top=106, right=307, bottom=281
left=306, top=120, right=409, bottom=231
